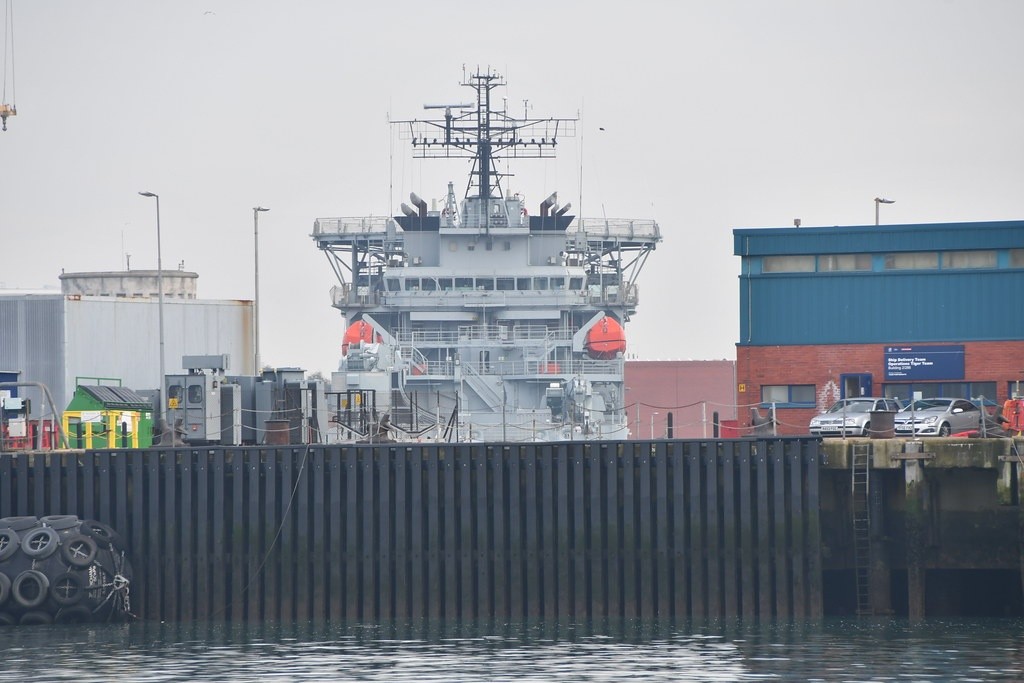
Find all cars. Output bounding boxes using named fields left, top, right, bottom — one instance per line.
left=810, top=397, right=903, bottom=437
left=895, top=397, right=981, bottom=437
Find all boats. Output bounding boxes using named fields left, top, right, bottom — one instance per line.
left=309, top=64, right=664, bottom=441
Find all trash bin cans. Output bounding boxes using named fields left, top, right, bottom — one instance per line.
left=264, top=420, right=291, bottom=445
left=868, top=410, right=899, bottom=439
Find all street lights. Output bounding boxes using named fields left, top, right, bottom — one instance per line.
left=875, top=197, right=896, bottom=225
left=252, top=206, right=270, bottom=376
left=138, top=191, right=167, bottom=427
